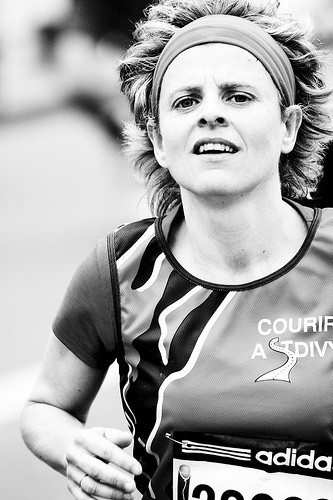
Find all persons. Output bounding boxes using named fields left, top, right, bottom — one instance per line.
left=21, top=2, right=333, bottom=500
